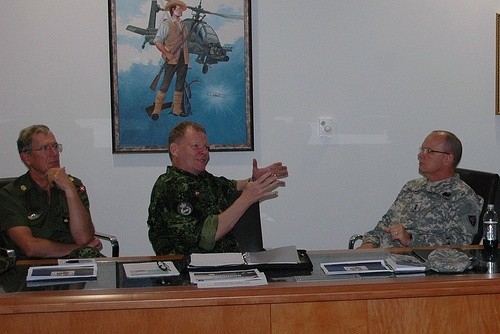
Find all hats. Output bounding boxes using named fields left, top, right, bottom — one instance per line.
left=428, top=247, right=471, bottom=272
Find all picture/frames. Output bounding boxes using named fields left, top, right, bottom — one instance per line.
left=108, top=0, right=254, bottom=154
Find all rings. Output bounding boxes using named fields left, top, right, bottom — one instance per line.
left=395, top=231, right=397, bottom=234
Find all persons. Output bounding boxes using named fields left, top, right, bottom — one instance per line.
left=356, top=130, right=484, bottom=249
left=0, top=125, right=109, bottom=259
left=147, top=121, right=288, bottom=256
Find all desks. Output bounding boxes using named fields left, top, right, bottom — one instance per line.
left=0, top=245, right=500, bottom=334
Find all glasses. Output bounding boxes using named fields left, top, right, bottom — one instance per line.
left=419, top=146, right=450, bottom=157
left=27, top=144, right=63, bottom=154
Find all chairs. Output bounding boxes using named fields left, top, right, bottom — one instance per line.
left=227, top=190, right=266, bottom=252
left=349, top=167, right=500, bottom=249
left=0, top=178, right=119, bottom=260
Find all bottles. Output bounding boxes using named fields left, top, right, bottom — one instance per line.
left=482, top=204, right=499, bottom=253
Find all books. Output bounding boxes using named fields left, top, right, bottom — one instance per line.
left=385, top=254, right=427, bottom=273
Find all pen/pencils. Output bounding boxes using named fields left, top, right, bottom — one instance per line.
left=65, top=260, right=92, bottom=262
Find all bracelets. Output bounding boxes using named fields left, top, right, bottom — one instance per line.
left=248, top=179, right=250, bottom=182
